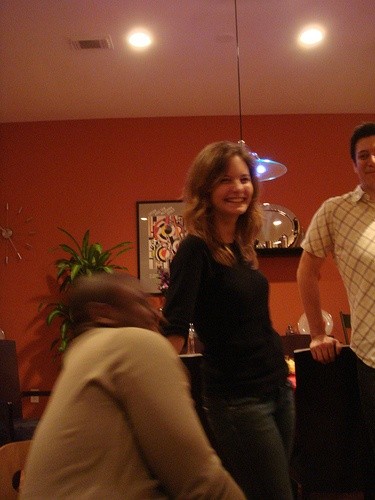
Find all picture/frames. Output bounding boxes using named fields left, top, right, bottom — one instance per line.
left=136, top=200, right=194, bottom=295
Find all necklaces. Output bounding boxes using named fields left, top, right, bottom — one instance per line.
left=224, top=239, right=234, bottom=244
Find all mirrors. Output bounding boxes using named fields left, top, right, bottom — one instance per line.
left=259, top=202, right=300, bottom=248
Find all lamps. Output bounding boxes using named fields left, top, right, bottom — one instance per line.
left=227, top=0, right=289, bottom=183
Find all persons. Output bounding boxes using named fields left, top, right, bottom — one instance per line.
left=297, top=121, right=375, bottom=500
left=161, top=140, right=295, bottom=500
left=18, top=275, right=247, bottom=500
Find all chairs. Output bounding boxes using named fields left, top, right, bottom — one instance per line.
left=288, top=343, right=375, bottom=500
left=0, top=440, right=32, bottom=500
left=0, top=338, right=52, bottom=445
